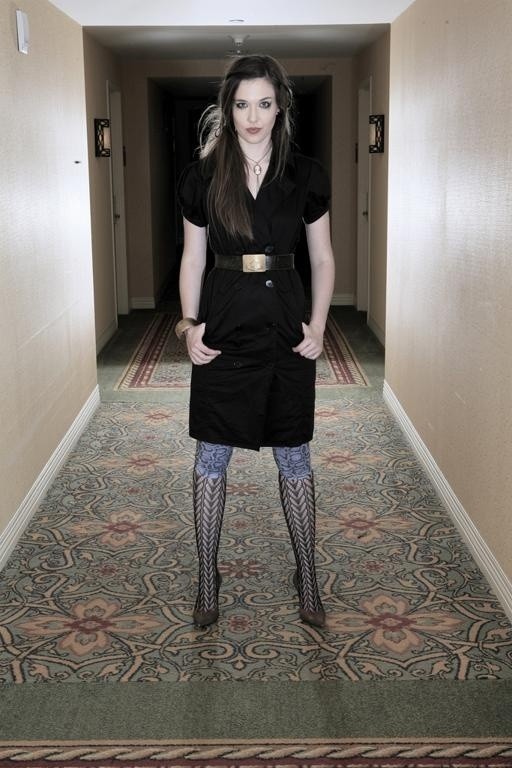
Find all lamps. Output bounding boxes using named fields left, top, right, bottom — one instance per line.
left=366, top=114, right=385, bottom=154
left=94, top=117, right=112, bottom=158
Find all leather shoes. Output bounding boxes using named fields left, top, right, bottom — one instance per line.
left=194, top=573, right=222, bottom=625
left=291, top=568, right=327, bottom=628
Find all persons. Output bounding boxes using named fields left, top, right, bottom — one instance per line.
left=173, top=54, right=338, bottom=630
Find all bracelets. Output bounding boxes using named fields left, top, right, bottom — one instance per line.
left=175, top=317, right=198, bottom=341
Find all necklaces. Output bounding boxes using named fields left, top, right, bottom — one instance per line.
left=240, top=146, right=272, bottom=186
